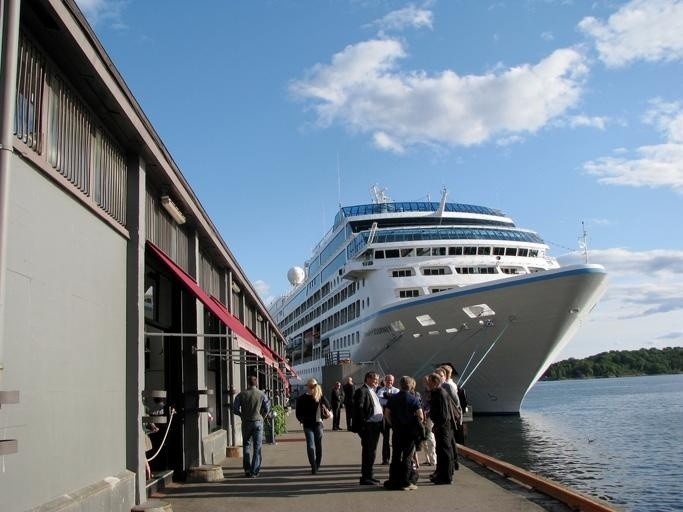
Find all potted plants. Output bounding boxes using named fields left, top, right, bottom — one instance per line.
left=263, top=404, right=286, bottom=443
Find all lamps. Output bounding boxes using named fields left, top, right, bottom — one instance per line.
left=258, top=314, right=263, bottom=321
left=232, top=281, right=241, bottom=294
left=162, top=194, right=187, bottom=225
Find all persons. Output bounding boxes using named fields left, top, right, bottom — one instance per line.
left=295, top=377, right=332, bottom=475
left=142, top=404, right=159, bottom=479
left=330, top=363, right=464, bottom=491
left=231, top=375, right=270, bottom=479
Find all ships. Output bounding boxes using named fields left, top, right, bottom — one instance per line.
left=265, top=184, right=608, bottom=414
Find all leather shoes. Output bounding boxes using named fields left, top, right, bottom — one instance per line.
left=371, top=479, right=380, bottom=482
left=360, top=481, right=374, bottom=485
left=382, top=461, right=388, bottom=464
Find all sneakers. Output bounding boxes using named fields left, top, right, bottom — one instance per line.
left=251, top=473, right=260, bottom=478
left=245, top=470, right=251, bottom=477
left=401, top=483, right=417, bottom=491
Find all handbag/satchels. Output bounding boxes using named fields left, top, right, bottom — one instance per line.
left=315, top=402, right=323, bottom=423
left=321, top=404, right=330, bottom=419
